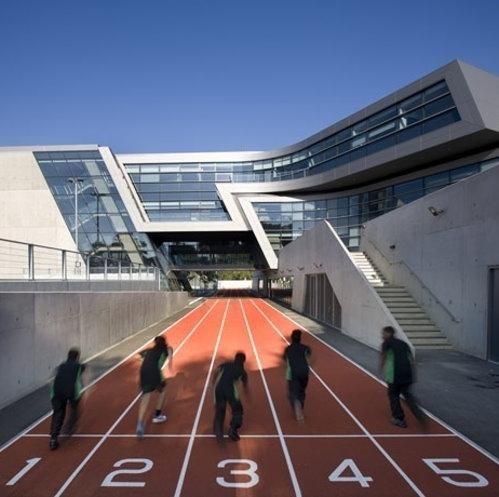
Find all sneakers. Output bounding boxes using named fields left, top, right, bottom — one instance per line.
left=135, top=423, right=146, bottom=439
left=49, top=439, right=59, bottom=450
left=151, top=414, right=167, bottom=423
left=389, top=417, right=408, bottom=428
left=226, top=426, right=241, bottom=440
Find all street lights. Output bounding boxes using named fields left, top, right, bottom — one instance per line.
left=68, top=174, right=86, bottom=274
left=90, top=191, right=108, bottom=256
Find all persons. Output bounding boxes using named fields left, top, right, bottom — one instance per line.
left=48, top=348, right=86, bottom=452
left=211, top=351, right=250, bottom=443
left=280, top=328, right=314, bottom=420
left=379, top=325, right=429, bottom=428
left=133, top=335, right=174, bottom=441
